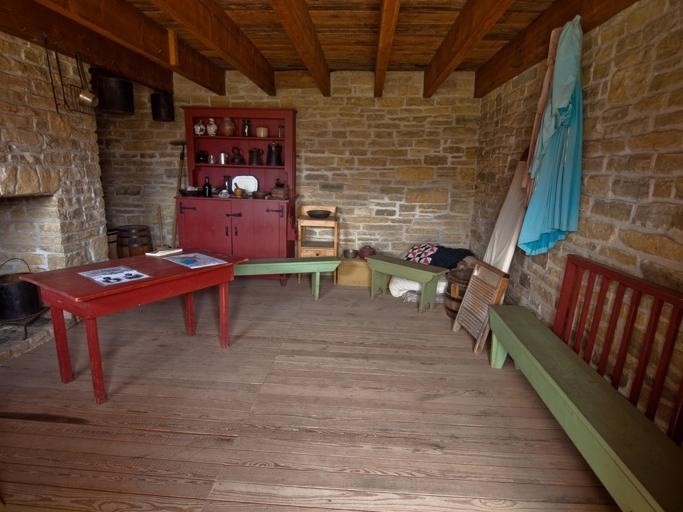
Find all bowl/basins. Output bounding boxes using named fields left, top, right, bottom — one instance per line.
left=306, top=210, right=331, bottom=219
left=343, top=249, right=358, bottom=258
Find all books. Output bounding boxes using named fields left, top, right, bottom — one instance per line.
left=144, top=248, right=182, bottom=256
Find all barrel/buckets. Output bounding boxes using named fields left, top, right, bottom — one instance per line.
left=445, top=269, right=472, bottom=318
left=116, top=224, right=153, bottom=257
left=0, top=257, right=42, bottom=319
left=107, top=228, right=119, bottom=260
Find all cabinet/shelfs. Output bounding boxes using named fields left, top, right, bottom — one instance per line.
left=176, top=105, right=392, bottom=288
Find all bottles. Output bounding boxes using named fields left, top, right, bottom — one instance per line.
left=202, top=176, right=212, bottom=198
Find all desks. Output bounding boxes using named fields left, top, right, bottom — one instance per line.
left=19, top=247, right=251, bottom=405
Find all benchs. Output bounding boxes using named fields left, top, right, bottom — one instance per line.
left=487, top=302, right=683, bottom=512
left=235, top=254, right=450, bottom=313
